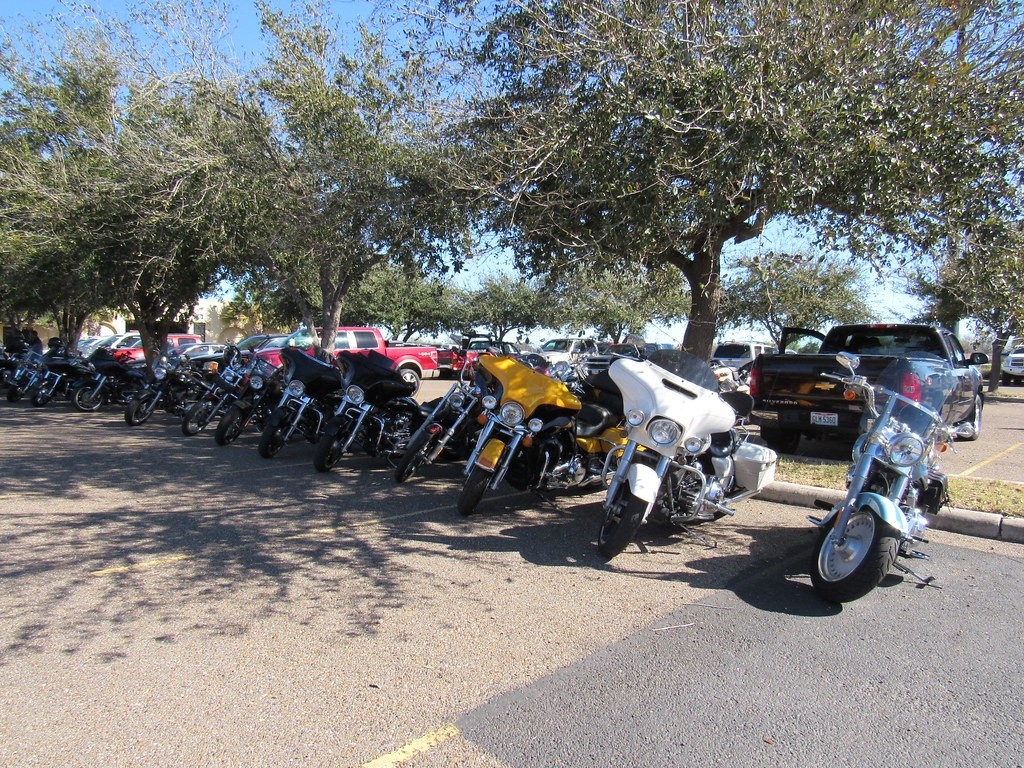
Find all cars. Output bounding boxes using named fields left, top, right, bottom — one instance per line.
left=532, top=337, right=603, bottom=377
left=110, top=333, right=205, bottom=363
left=452, top=337, right=526, bottom=378
left=125, top=343, right=231, bottom=377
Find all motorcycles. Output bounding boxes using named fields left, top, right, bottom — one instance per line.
left=806, top=351, right=975, bottom=607
left=1, top=333, right=651, bottom=516
left=589, top=348, right=777, bottom=560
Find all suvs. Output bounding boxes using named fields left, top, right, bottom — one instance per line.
left=1001, top=345, right=1024, bottom=385
left=710, top=340, right=800, bottom=377
left=189, top=332, right=292, bottom=383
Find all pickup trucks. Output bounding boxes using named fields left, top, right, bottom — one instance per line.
left=740, top=322, right=991, bottom=442
left=255, top=327, right=441, bottom=401
